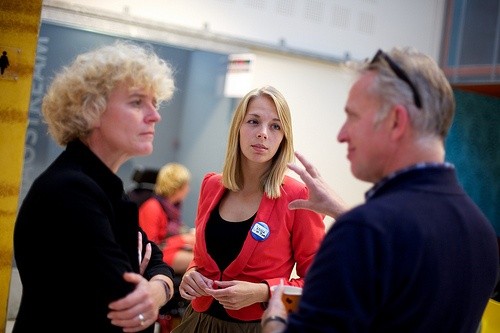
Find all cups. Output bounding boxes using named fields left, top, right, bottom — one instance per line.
left=270, top=285, right=303, bottom=314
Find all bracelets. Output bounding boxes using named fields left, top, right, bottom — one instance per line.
left=263, top=316, right=287, bottom=327
left=150, top=274, right=174, bottom=301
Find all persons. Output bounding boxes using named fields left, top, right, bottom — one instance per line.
left=262, top=46, right=500, bottom=333
left=138, top=162, right=196, bottom=333
left=12, top=38, right=177, bottom=333
left=169, top=85, right=325, bottom=333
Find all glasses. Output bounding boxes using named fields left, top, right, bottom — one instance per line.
left=369, top=48, right=423, bottom=109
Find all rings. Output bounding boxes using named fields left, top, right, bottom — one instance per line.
left=139, top=315, right=144, bottom=320
